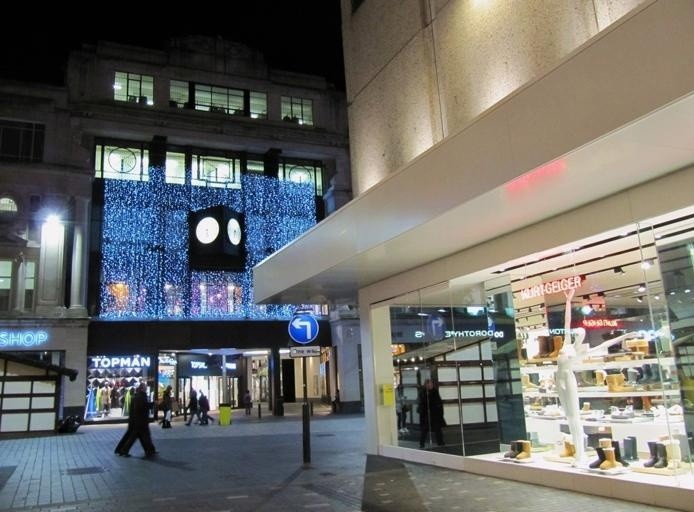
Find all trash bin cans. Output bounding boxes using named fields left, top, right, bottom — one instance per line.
left=219, top=403, right=231, bottom=425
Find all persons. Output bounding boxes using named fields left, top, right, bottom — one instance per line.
left=554, top=286, right=640, bottom=463
left=159, top=384, right=173, bottom=429
left=393, top=383, right=409, bottom=433
left=116, top=382, right=160, bottom=458
left=99, top=381, right=112, bottom=416
left=330, top=389, right=340, bottom=414
left=112, top=386, right=159, bottom=455
left=242, top=389, right=251, bottom=416
left=416, top=378, right=447, bottom=449
left=181, top=387, right=215, bottom=426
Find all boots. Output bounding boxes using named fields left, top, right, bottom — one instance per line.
left=503, top=335, right=683, bottom=470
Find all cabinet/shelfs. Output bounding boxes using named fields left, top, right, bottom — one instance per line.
left=90, top=368, right=143, bottom=419
left=518, top=328, right=694, bottom=464
